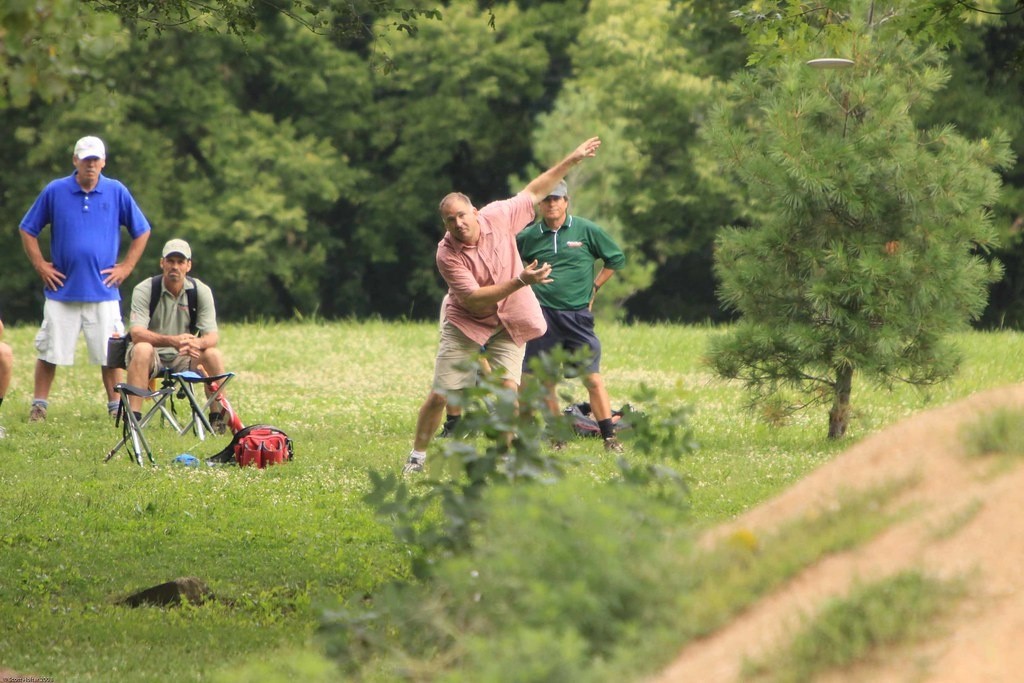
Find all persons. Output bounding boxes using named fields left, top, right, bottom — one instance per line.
left=514, top=178, right=626, bottom=457
left=122, top=237, right=226, bottom=434
left=400, top=135, right=601, bottom=474
left=18, top=136, right=153, bottom=426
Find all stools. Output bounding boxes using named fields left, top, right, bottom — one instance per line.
left=172, top=370, right=234, bottom=439
left=103, top=383, right=175, bottom=468
left=141, top=367, right=183, bottom=432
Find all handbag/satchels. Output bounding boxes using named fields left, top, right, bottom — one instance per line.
left=206, top=424, right=294, bottom=468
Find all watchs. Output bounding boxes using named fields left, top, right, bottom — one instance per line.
left=593, top=283, right=600, bottom=292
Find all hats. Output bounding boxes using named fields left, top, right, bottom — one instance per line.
left=549, top=178, right=568, bottom=197
left=75, top=136, right=105, bottom=160
left=162, top=239, right=191, bottom=259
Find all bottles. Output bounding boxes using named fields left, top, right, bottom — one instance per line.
left=107, top=326, right=126, bottom=369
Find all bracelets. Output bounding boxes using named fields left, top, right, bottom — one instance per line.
left=517, top=275, right=528, bottom=286
left=200, top=337, right=207, bottom=349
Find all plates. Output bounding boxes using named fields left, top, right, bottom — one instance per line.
left=805, top=55, right=856, bottom=71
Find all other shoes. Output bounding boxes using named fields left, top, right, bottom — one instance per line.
left=552, top=439, right=567, bottom=453
left=604, top=437, right=625, bottom=457
left=0, top=426, right=7, bottom=440
left=435, top=422, right=469, bottom=441
left=210, top=420, right=227, bottom=436
left=402, top=456, right=427, bottom=478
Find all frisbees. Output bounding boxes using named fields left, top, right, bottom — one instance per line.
left=806, top=58, right=855, bottom=69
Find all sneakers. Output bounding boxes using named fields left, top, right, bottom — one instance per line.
left=28, top=404, right=47, bottom=424
left=108, top=407, right=119, bottom=418
